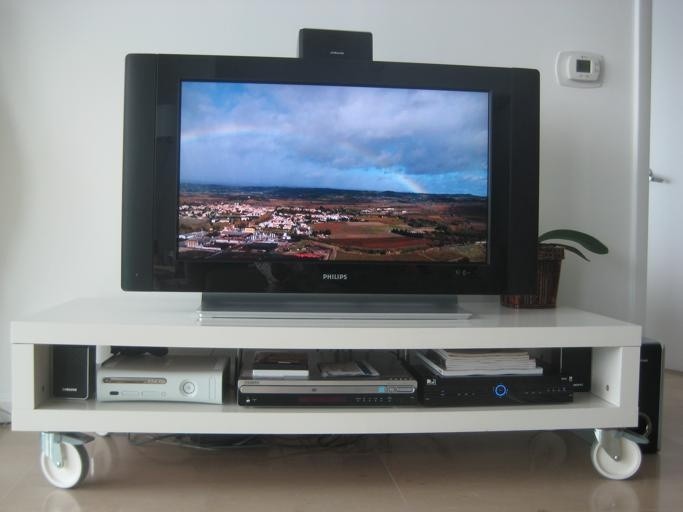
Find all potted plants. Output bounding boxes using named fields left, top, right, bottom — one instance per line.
left=501, top=229, right=608, bottom=309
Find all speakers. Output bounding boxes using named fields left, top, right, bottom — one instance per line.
left=624, top=338, right=665, bottom=454
left=51, top=345, right=95, bottom=399
left=553, top=347, right=591, bottom=392
left=298, top=28, right=372, bottom=60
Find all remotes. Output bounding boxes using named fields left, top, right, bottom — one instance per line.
left=111, top=346, right=169, bottom=357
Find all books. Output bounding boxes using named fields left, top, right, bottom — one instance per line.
left=416, top=349, right=543, bottom=376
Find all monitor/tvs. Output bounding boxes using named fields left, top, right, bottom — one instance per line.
left=121, top=52, right=540, bottom=319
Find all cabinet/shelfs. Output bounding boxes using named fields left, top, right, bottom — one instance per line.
left=10, top=295, right=650, bottom=488
